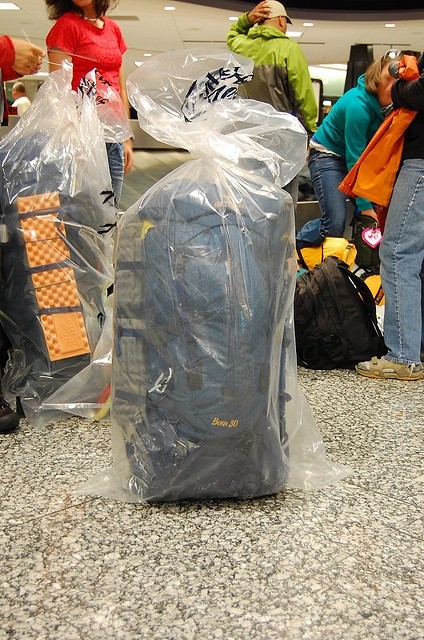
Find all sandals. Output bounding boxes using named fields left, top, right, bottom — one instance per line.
left=355, top=356, right=424, bottom=380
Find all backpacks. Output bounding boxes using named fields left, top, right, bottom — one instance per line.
left=295, top=257, right=387, bottom=369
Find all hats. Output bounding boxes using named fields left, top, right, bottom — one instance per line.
left=260, top=0, right=293, bottom=25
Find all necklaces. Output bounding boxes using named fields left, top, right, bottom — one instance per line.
left=79, top=14, right=98, bottom=22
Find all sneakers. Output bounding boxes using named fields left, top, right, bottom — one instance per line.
left=0, top=396, right=19, bottom=431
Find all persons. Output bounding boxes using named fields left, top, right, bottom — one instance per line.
left=45, top=0, right=133, bottom=209
left=228, top=1, right=317, bottom=209
left=355, top=51, right=424, bottom=380
left=12, top=83, right=31, bottom=115
left=0, top=36, right=47, bottom=434
left=295, top=49, right=400, bottom=248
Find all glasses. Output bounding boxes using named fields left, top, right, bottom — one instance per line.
left=381, top=49, right=401, bottom=78
